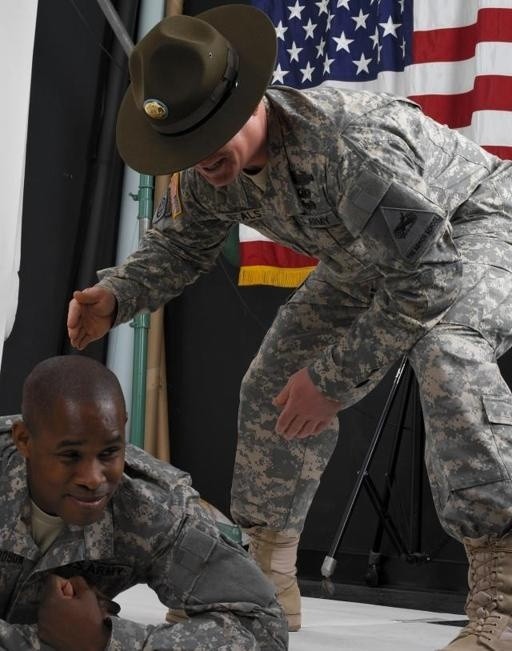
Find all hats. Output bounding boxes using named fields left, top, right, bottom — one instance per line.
left=113, top=3, right=281, bottom=179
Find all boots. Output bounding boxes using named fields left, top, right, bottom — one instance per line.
left=439, top=530, right=512, bottom=650
left=164, top=524, right=305, bottom=633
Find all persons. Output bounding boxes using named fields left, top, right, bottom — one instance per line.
left=63, top=84, right=511, bottom=651
left=2, top=354, right=290, bottom=651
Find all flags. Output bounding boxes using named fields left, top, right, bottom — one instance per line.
left=231, top=2, right=512, bottom=291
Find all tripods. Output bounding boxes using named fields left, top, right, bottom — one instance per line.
left=321, top=354, right=450, bottom=578
